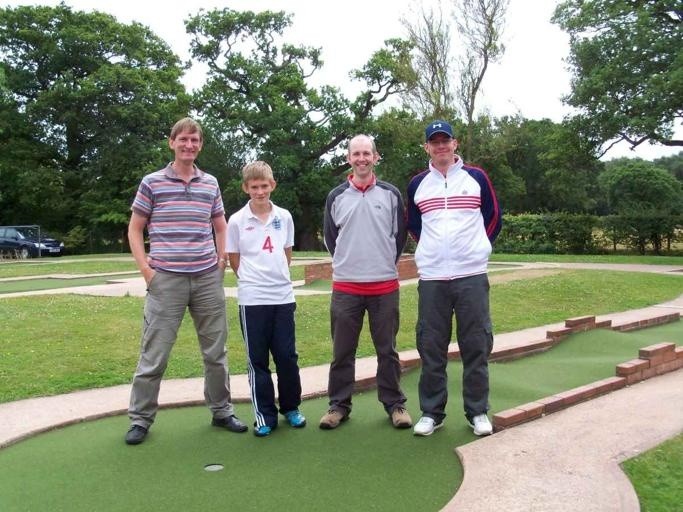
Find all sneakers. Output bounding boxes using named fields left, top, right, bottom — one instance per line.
left=413, top=416, right=444, bottom=436
left=390, top=407, right=413, bottom=428
left=466, top=413, right=493, bottom=436
left=254, top=426, right=273, bottom=437
left=211, top=415, right=248, bottom=432
left=125, top=425, right=147, bottom=445
left=284, top=409, right=306, bottom=427
left=319, top=409, right=351, bottom=429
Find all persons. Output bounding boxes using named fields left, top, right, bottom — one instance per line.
left=406, top=119, right=505, bottom=438
left=123, top=114, right=247, bottom=442
left=319, top=134, right=413, bottom=428
left=225, top=162, right=309, bottom=438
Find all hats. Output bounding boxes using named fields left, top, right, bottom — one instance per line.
left=425, top=120, right=454, bottom=143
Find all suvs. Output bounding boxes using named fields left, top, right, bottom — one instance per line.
left=0, top=225, right=65, bottom=258
